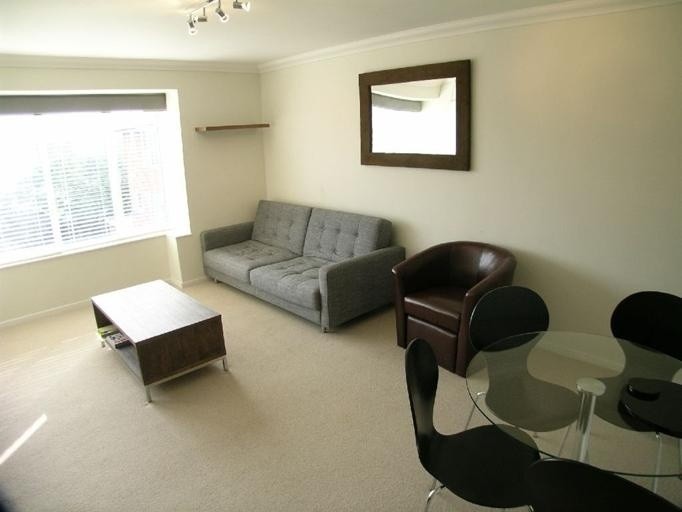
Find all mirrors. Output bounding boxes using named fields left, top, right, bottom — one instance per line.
left=358, top=59, right=470, bottom=170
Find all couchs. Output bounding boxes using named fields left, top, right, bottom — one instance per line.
left=200, top=200, right=406, bottom=332
left=392, top=240, right=516, bottom=378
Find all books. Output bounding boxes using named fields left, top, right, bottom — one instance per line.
left=106, top=332, right=130, bottom=348
left=98, top=324, right=119, bottom=337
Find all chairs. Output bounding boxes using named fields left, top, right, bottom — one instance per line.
left=579, top=290, right=682, bottom=493
left=405, top=339, right=541, bottom=510
left=466, top=284, right=582, bottom=458
left=529, top=459, right=680, bottom=511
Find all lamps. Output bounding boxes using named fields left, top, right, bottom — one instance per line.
left=187, top=0, right=253, bottom=33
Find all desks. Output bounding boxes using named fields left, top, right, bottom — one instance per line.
left=462, top=331, right=681, bottom=491
